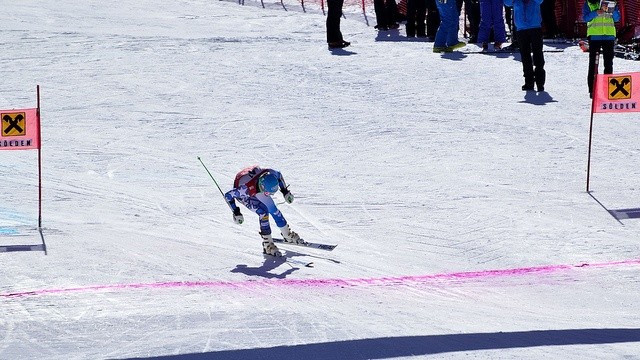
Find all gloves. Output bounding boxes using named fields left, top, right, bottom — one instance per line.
left=232, top=207, right=244, bottom=224
left=280, top=188, right=294, bottom=204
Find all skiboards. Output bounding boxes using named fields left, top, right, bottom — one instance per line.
left=263, top=238, right=338, bottom=267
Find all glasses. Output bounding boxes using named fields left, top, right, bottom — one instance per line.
left=258, top=172, right=278, bottom=193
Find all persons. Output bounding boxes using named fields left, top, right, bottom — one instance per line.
left=477, top=0, right=507, bottom=49
left=373, top=0, right=399, bottom=30
left=224, top=165, right=299, bottom=256
left=582, top=0, right=622, bottom=98
left=463, top=0, right=494, bottom=43
left=433, top=0, right=466, bottom=52
left=426, top=0, right=441, bottom=42
left=503, top=0, right=545, bottom=90
left=406, top=0, right=427, bottom=38
left=326, top=0, right=350, bottom=48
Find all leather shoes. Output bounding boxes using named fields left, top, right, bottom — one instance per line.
left=328, top=41, right=350, bottom=50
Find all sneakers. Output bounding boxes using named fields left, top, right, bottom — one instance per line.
left=389, top=23, right=398, bottom=29
left=280, top=231, right=300, bottom=241
left=522, top=84, right=534, bottom=91
left=538, top=85, right=544, bottom=93
left=433, top=44, right=452, bottom=53
left=447, top=41, right=466, bottom=48
left=375, top=24, right=386, bottom=30
left=262, top=241, right=279, bottom=253
